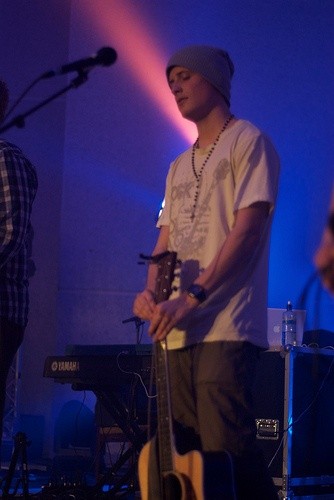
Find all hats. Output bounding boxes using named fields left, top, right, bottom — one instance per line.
left=165, top=45, right=234, bottom=106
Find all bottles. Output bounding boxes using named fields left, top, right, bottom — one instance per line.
left=282, top=301, right=296, bottom=347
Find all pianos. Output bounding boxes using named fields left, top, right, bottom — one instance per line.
left=43, top=356, right=156, bottom=384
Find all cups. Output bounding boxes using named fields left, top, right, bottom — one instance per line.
left=254, top=418, right=281, bottom=441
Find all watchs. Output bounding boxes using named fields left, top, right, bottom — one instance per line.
left=187, top=283, right=208, bottom=302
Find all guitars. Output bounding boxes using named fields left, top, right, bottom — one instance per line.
left=137, top=250, right=235, bottom=500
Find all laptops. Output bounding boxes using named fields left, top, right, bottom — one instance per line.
left=268, top=308, right=306, bottom=345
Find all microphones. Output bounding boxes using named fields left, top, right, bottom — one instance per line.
left=40, top=46, right=117, bottom=80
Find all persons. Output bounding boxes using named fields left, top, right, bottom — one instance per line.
left=133, top=44, right=281, bottom=499
left=0, top=80, right=38, bottom=424
